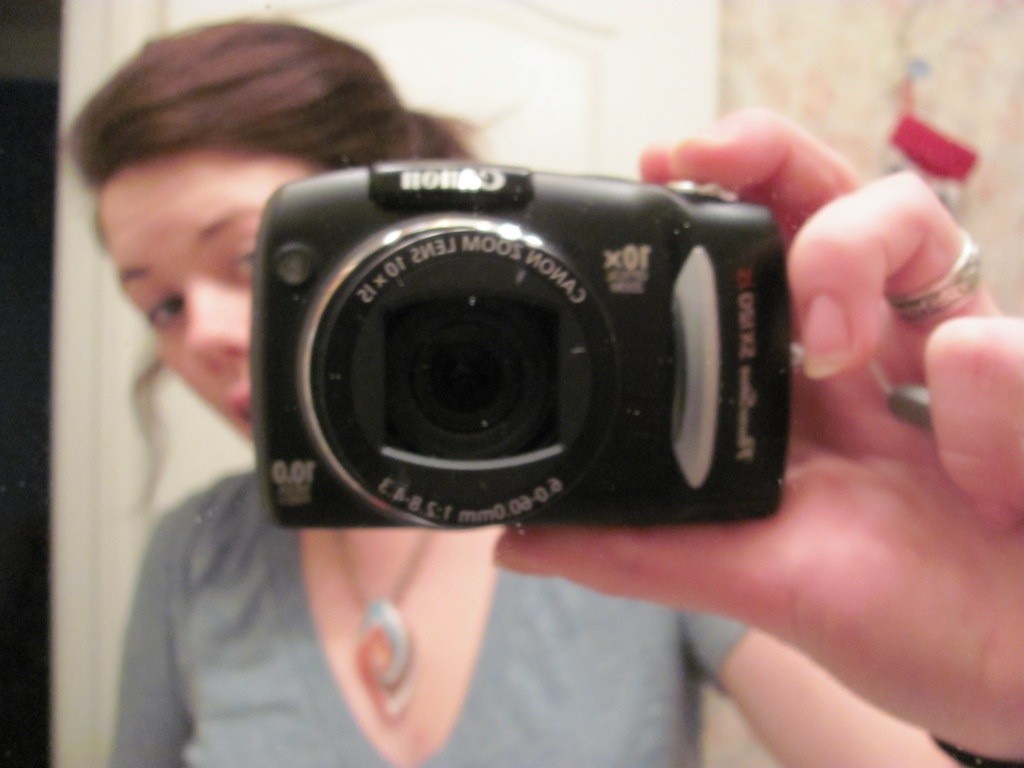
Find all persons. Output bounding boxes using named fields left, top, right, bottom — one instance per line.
left=61, top=21, right=1024, bottom=768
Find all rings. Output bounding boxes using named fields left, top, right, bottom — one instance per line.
left=890, top=237, right=983, bottom=321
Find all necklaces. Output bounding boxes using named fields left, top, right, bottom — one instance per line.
left=305, top=517, right=449, bottom=702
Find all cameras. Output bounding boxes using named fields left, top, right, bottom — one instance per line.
left=249, top=156, right=793, bottom=527
